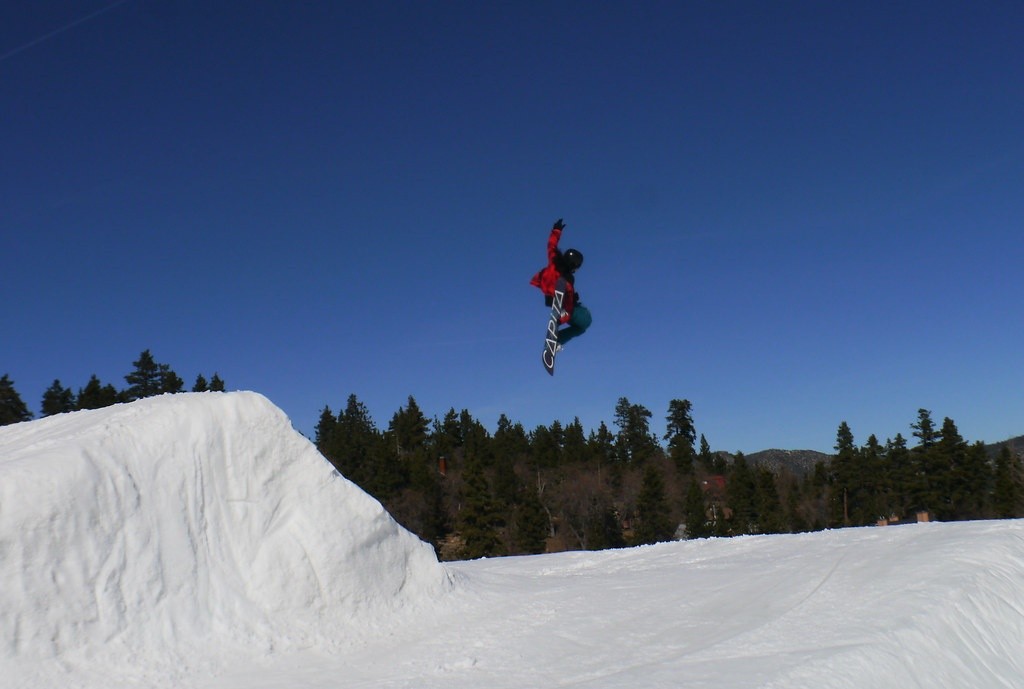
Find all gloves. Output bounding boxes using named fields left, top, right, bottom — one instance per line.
left=553, top=219, right=565, bottom=229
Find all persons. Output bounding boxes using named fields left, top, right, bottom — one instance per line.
left=529, top=217, right=592, bottom=351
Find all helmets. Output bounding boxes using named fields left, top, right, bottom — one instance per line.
left=564, top=249, right=582, bottom=269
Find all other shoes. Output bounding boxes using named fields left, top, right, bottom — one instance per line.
left=555, top=343, right=562, bottom=352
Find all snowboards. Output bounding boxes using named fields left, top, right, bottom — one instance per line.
left=541, top=277, right=568, bottom=376
